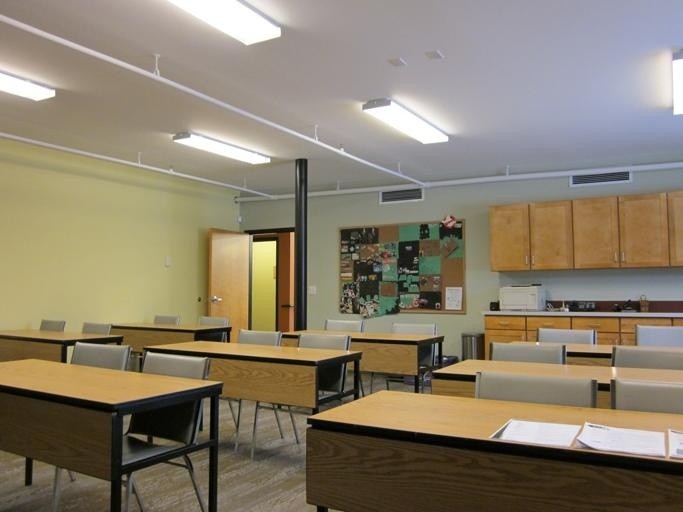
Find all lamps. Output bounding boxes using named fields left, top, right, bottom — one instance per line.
left=361, top=98, right=448, bottom=145
left=166, top=0, right=281, bottom=47
left=171, top=131, right=271, bottom=165
left=671, top=51, right=682, bottom=116
left=0, top=72, right=56, bottom=102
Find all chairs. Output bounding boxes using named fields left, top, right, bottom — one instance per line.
left=369, top=324, right=438, bottom=394
left=225, top=328, right=350, bottom=458
left=323, top=319, right=365, bottom=398
left=39, top=315, right=228, bottom=335
left=53, top=341, right=212, bottom=512
left=430, top=328, right=683, bottom=407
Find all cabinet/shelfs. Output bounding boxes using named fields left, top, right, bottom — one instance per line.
left=485, top=317, right=682, bottom=361
left=488, top=199, right=574, bottom=272
left=571, top=192, right=670, bottom=270
left=667, top=191, right=683, bottom=268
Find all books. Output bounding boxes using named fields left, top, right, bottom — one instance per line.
left=490, top=419, right=683, bottom=461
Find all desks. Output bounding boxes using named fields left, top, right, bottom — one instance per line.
left=0, top=358, right=223, bottom=512
left=114, top=321, right=233, bottom=354
left=305, top=389, right=682, bottom=510
left=0, top=329, right=123, bottom=363
left=144, top=341, right=362, bottom=414
left=282, top=330, right=443, bottom=394
